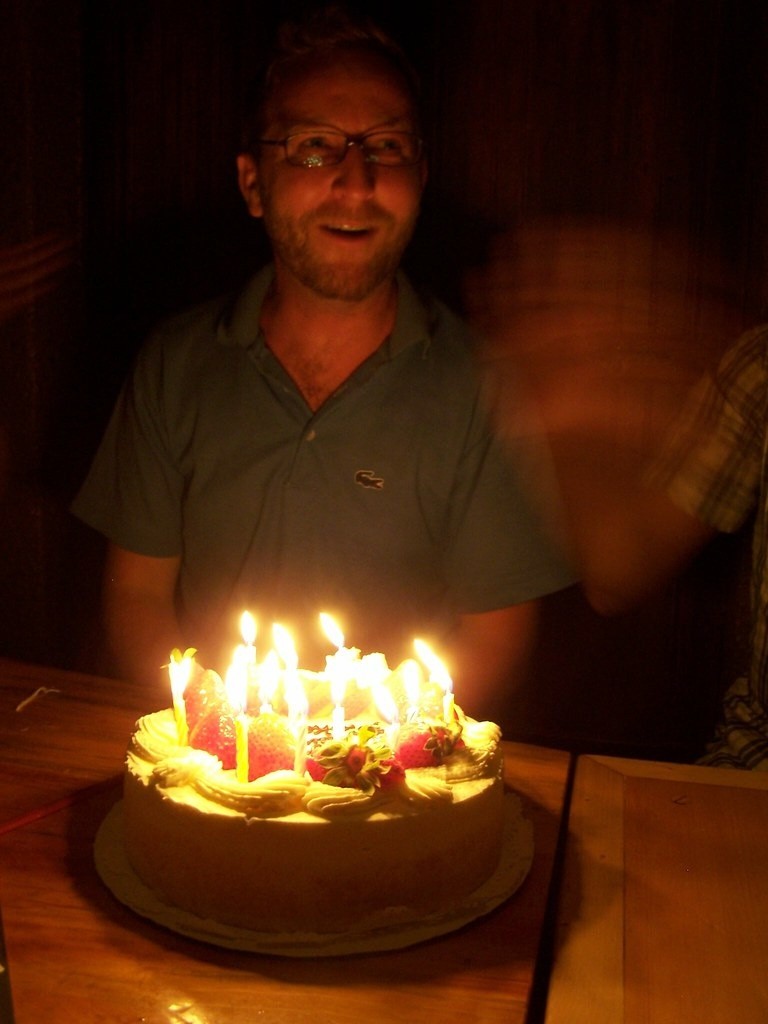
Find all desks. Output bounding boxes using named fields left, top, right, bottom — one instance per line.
left=541, top=755, right=768, bottom=1024
left=0, top=660, right=572, bottom=1024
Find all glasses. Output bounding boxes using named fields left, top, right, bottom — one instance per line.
left=259, top=130, right=429, bottom=167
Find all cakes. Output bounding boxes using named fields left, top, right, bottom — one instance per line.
left=122, top=646, right=505, bottom=935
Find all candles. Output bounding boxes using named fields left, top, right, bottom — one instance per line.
left=166, top=607, right=455, bottom=779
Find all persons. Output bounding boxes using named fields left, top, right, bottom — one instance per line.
left=484, top=323, right=768, bottom=776
left=71, top=40, right=575, bottom=722
